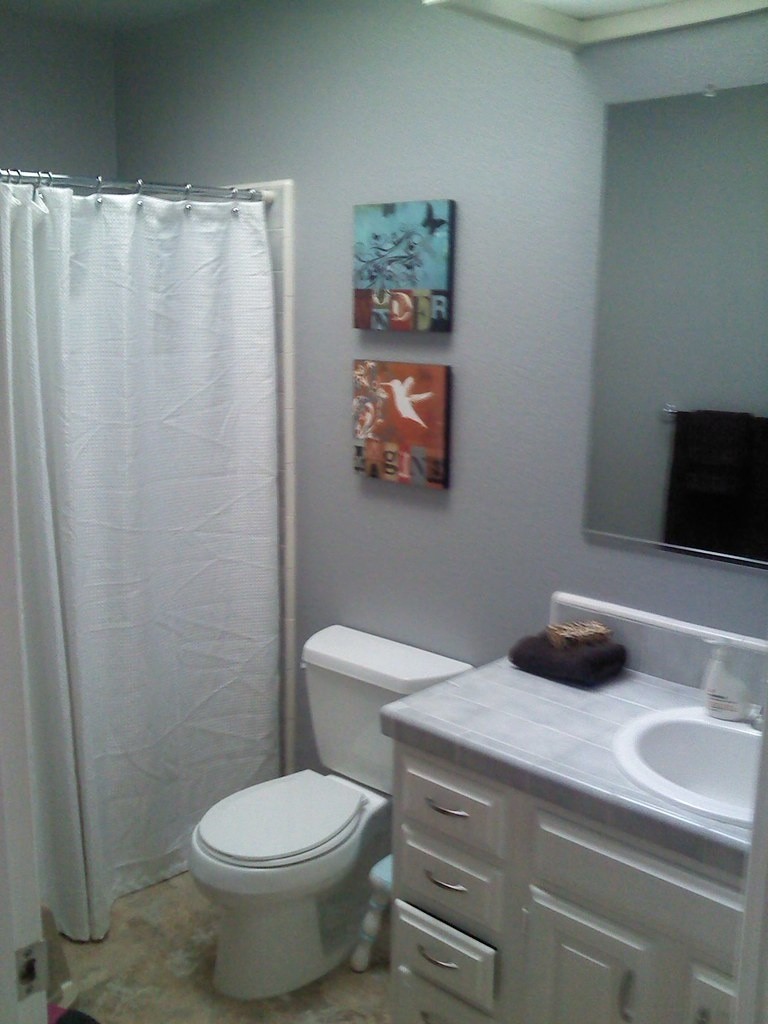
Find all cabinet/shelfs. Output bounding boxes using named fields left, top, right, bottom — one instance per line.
left=387, top=741, right=768, bottom=1024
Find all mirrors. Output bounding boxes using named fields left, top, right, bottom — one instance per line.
left=578, top=84, right=768, bottom=573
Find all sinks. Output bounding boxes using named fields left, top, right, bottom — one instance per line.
left=611, top=704, right=764, bottom=829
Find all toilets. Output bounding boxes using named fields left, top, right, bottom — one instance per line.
left=190, top=620, right=478, bottom=1001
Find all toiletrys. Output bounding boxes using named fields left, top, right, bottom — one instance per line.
left=701, top=638, right=749, bottom=721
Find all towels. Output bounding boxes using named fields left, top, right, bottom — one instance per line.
left=508, top=632, right=627, bottom=690
